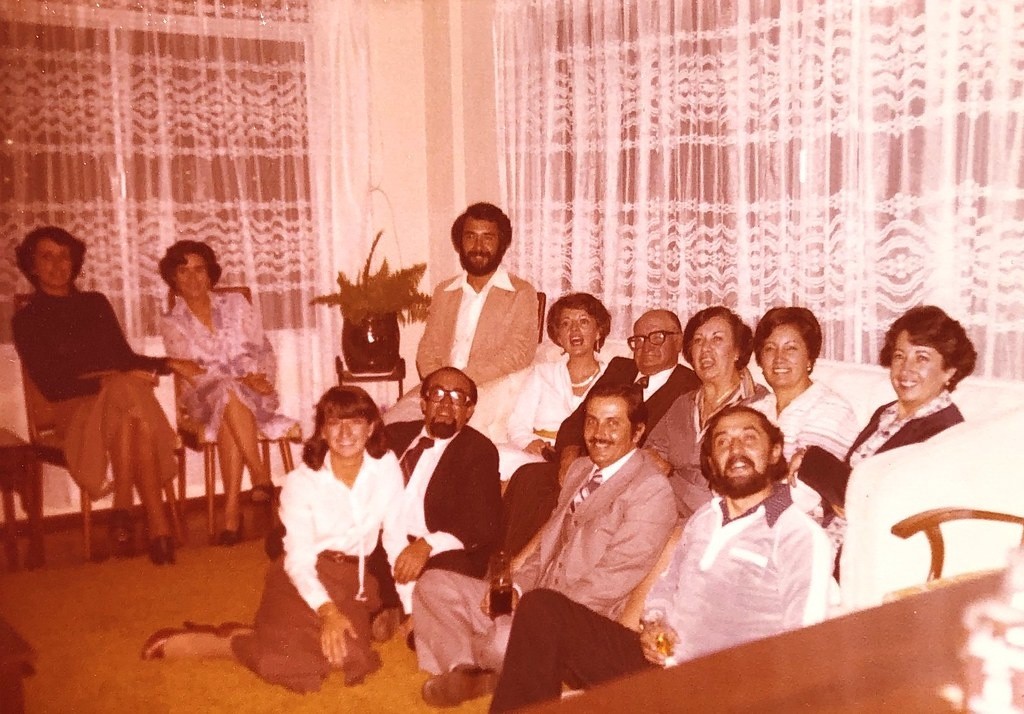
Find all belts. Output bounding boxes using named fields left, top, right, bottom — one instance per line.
left=320, top=550, right=359, bottom=564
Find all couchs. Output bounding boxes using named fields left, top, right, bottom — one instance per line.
left=468, top=338, right=1024, bottom=610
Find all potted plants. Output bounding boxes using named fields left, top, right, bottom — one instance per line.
left=308, top=231, right=432, bottom=374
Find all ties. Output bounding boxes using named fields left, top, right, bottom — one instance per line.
left=633, top=375, right=649, bottom=389
left=565, top=469, right=602, bottom=515
left=401, top=437, right=434, bottom=488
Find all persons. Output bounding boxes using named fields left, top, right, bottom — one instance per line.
left=490, top=404, right=834, bottom=714
left=12, top=225, right=206, bottom=567
left=143, top=385, right=404, bottom=693
left=498, top=293, right=612, bottom=496
left=412, top=382, right=677, bottom=707
left=641, top=307, right=770, bottom=513
left=492, top=309, right=703, bottom=578
left=381, top=202, right=539, bottom=425
left=788, top=305, right=977, bottom=587
left=746, top=307, right=859, bottom=462
left=159, top=239, right=281, bottom=546
left=368, top=367, right=500, bottom=650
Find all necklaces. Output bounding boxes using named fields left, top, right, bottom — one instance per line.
left=716, top=380, right=739, bottom=403
left=731, top=498, right=747, bottom=513
left=572, top=368, right=599, bottom=387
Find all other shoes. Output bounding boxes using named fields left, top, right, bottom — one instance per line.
left=151, top=533, right=175, bottom=565
left=249, top=481, right=273, bottom=504
left=109, top=510, right=134, bottom=550
left=422, top=665, right=496, bottom=708
left=369, top=603, right=410, bottom=642
left=220, top=510, right=244, bottom=546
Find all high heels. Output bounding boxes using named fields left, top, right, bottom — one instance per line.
left=142, top=620, right=217, bottom=661
left=217, top=623, right=258, bottom=643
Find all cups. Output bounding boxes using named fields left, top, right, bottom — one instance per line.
left=639, top=604, right=678, bottom=669
left=490, top=563, right=512, bottom=619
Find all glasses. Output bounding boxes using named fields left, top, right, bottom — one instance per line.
left=628, top=331, right=677, bottom=351
left=425, top=385, right=471, bottom=406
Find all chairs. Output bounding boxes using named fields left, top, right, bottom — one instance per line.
left=168, top=287, right=299, bottom=538
left=14, top=292, right=185, bottom=562
